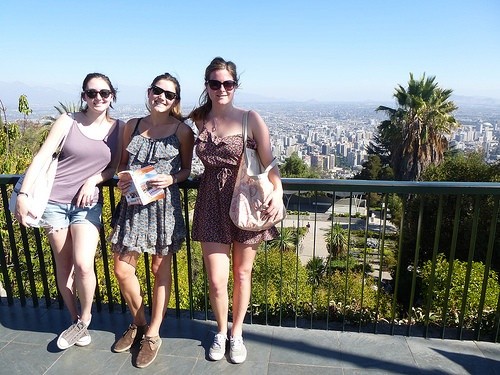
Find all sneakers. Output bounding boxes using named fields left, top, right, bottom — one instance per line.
left=56, top=314, right=92, bottom=349
left=136, top=335, right=162, bottom=367
left=230, top=335, right=247, bottom=363
left=112, top=320, right=149, bottom=352
left=74, top=329, right=91, bottom=346
left=209, top=333, right=228, bottom=360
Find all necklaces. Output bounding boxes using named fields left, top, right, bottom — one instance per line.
left=211, top=105, right=233, bottom=132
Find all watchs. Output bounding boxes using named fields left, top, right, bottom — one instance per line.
left=172, top=174, right=178, bottom=184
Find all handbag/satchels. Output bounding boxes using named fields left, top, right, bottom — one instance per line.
left=9, top=112, right=74, bottom=225
left=229, top=109, right=286, bottom=231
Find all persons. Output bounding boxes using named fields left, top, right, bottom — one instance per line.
left=109, top=73, right=195, bottom=369
left=16, top=73, right=127, bottom=350
left=184, top=57, right=283, bottom=364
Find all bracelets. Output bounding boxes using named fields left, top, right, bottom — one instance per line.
left=17, top=191, right=29, bottom=198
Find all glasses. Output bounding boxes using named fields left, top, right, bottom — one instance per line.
left=206, top=80, right=237, bottom=91
left=152, top=85, right=178, bottom=100
left=86, top=89, right=112, bottom=99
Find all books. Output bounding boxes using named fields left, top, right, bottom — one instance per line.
left=117, top=165, right=164, bottom=206
